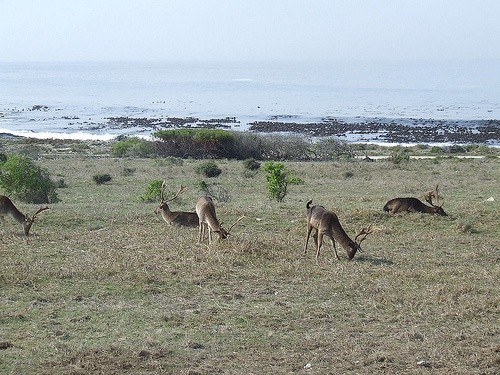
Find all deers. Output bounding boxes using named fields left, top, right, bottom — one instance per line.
left=195, top=196, right=245, bottom=246
left=0, top=194, right=51, bottom=236
left=382, top=185, right=448, bottom=217
left=302, top=199, right=373, bottom=262
left=155, top=179, right=199, bottom=228
